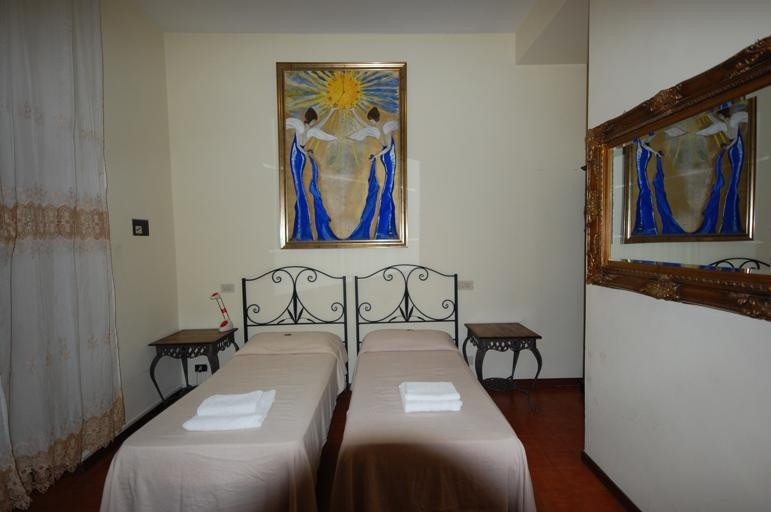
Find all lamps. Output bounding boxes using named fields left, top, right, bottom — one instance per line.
left=211, top=291, right=234, bottom=332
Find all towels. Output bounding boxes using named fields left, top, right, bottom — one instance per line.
left=183, top=388, right=276, bottom=431
left=402, top=380, right=460, bottom=401
left=196, top=390, right=262, bottom=415
left=399, top=383, right=464, bottom=412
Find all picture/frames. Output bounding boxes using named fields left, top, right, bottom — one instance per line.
left=623, top=98, right=757, bottom=243
left=275, top=58, right=410, bottom=249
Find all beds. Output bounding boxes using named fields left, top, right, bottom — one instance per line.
left=329, top=263, right=539, bottom=511
left=100, top=264, right=350, bottom=511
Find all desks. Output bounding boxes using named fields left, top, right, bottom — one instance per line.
left=462, top=321, right=544, bottom=406
left=148, top=328, right=241, bottom=406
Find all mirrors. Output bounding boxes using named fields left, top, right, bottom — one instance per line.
left=579, top=32, right=771, bottom=323
